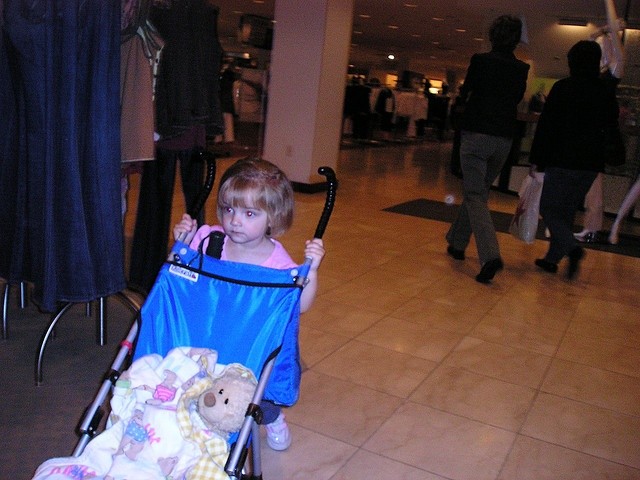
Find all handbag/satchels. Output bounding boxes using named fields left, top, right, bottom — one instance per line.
left=509, top=171, right=545, bottom=243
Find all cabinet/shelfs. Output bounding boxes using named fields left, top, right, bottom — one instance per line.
left=451, top=109, right=640, bottom=218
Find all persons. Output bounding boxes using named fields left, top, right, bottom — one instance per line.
left=531, top=42, right=620, bottom=282
left=446, top=14, right=530, bottom=282
left=608, top=177, right=640, bottom=245
left=546, top=1, right=627, bottom=240
left=173, top=159, right=325, bottom=451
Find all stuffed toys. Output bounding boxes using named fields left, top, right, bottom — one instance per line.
left=193, top=374, right=259, bottom=440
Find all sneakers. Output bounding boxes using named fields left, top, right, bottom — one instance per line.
left=476, top=259, right=503, bottom=284
left=264, top=410, right=291, bottom=451
left=447, top=246, right=465, bottom=260
left=535, top=258, right=558, bottom=273
left=563, top=249, right=585, bottom=280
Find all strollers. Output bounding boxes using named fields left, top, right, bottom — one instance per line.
left=70, top=149, right=337, bottom=480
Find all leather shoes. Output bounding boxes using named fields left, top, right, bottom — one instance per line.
left=574, top=232, right=593, bottom=243
